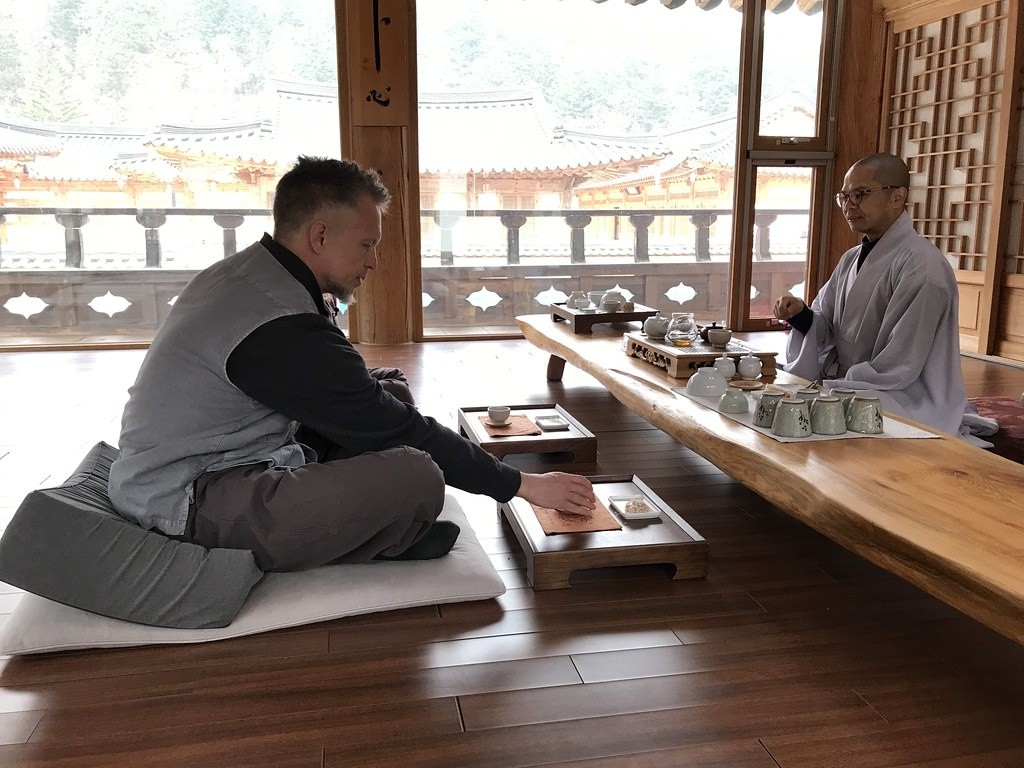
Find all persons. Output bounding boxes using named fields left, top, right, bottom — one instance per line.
left=773, top=154, right=999, bottom=437
left=108, top=154, right=597, bottom=572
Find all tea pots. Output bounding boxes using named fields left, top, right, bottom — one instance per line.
left=700, top=322, right=723, bottom=343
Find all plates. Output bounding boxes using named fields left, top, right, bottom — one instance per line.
left=730, top=381, right=763, bottom=389
left=608, top=493, right=661, bottom=520
left=485, top=419, right=512, bottom=427
left=534, top=415, right=570, bottom=429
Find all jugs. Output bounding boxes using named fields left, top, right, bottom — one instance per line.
left=667, top=312, right=698, bottom=346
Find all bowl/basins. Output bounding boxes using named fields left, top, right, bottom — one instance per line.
left=709, top=328, right=732, bottom=347
left=718, top=388, right=749, bottom=413
left=685, top=367, right=729, bottom=396
left=567, top=289, right=634, bottom=313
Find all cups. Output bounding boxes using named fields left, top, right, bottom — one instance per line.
left=714, top=353, right=736, bottom=381
left=738, top=351, right=761, bottom=380
left=644, top=313, right=669, bottom=339
left=752, top=385, right=884, bottom=436
left=623, top=302, right=634, bottom=313
left=488, top=406, right=511, bottom=422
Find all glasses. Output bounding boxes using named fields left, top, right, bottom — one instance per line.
left=833, top=185, right=900, bottom=207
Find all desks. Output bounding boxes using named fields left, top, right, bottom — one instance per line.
left=454, top=395, right=601, bottom=472
left=504, top=469, right=718, bottom=594
left=509, top=298, right=1024, bottom=670
